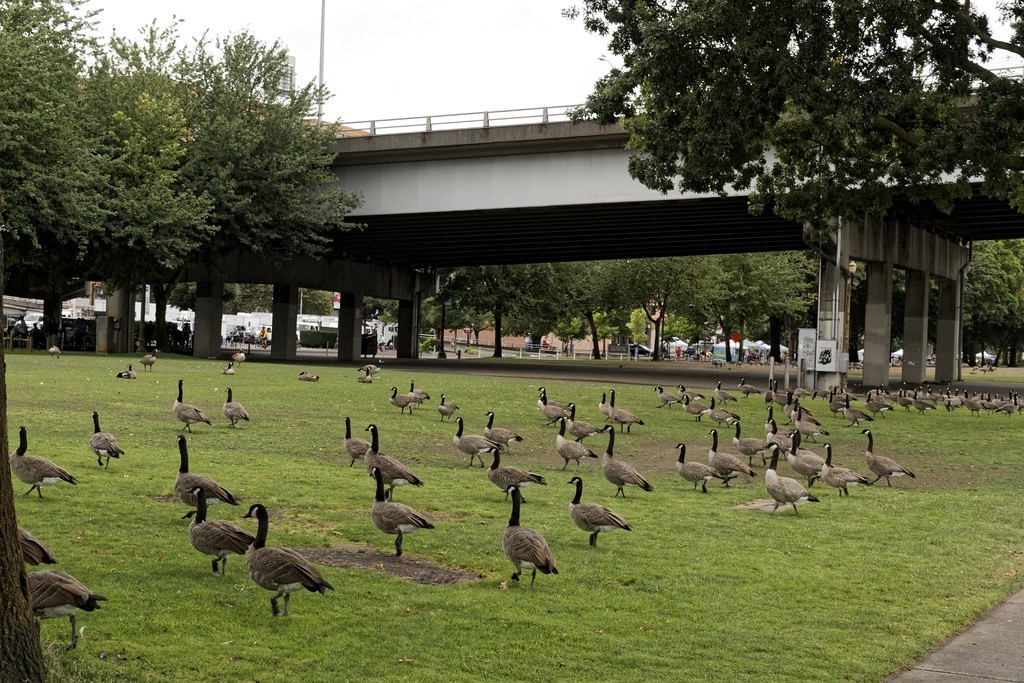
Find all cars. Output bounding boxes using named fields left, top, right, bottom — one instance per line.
left=629, top=343, right=653, bottom=358
left=542, top=340, right=557, bottom=353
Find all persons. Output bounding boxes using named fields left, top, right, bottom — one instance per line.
left=258, top=327, right=268, bottom=349
left=16, top=315, right=27, bottom=348
left=146, top=321, right=155, bottom=346
left=676, top=347, right=682, bottom=359
left=31, top=323, right=39, bottom=333
left=747, top=349, right=755, bottom=362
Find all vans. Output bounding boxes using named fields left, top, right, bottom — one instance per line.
left=14, top=310, right=73, bottom=332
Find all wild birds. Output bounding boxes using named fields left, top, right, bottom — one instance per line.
left=172, top=379, right=212, bottom=433
left=89, top=411, right=125, bottom=469
left=174, top=434, right=243, bottom=521
left=17, top=525, right=58, bottom=566
left=242, top=504, right=335, bottom=618
left=369, top=467, right=435, bottom=557
left=356, top=363, right=1024, bottom=588
left=230, top=348, right=245, bottom=367
left=140, top=348, right=158, bottom=372
left=221, top=362, right=235, bottom=375
left=364, top=424, right=424, bottom=501
left=9, top=425, right=81, bottom=499
left=115, top=364, right=137, bottom=379
left=48, top=344, right=62, bottom=359
left=343, top=416, right=385, bottom=467
left=299, top=371, right=320, bottom=382
left=223, top=387, right=250, bottom=428
left=24, top=568, right=109, bottom=652
left=185, top=486, right=256, bottom=576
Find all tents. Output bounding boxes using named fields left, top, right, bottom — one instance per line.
left=663, top=336, right=688, bottom=353
left=890, top=348, right=903, bottom=360
left=975, top=351, right=993, bottom=363
left=713, top=340, right=789, bottom=360
